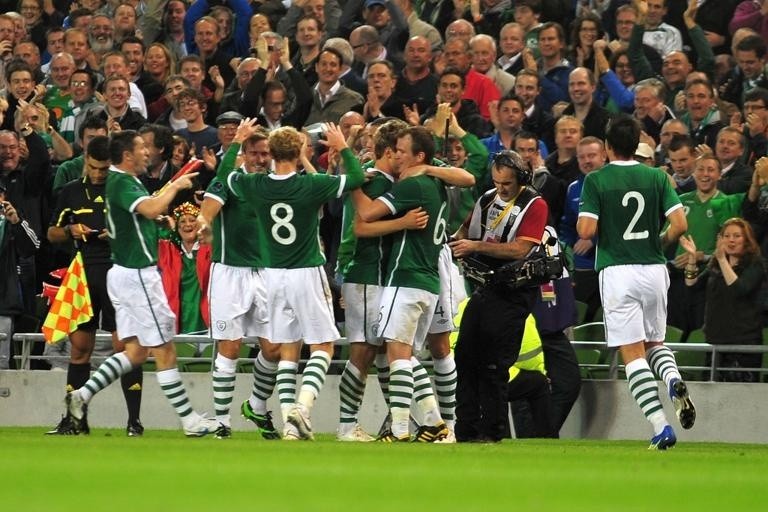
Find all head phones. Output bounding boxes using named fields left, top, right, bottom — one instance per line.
left=491, top=154, right=531, bottom=186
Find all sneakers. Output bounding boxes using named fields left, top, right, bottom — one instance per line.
left=288, top=407, right=315, bottom=441
left=180, top=418, right=223, bottom=437
left=213, top=423, right=232, bottom=440
left=378, top=414, right=392, bottom=435
left=282, top=430, right=306, bottom=441
left=240, top=400, right=280, bottom=440
left=335, top=425, right=376, bottom=442
left=66, top=390, right=91, bottom=435
left=434, top=429, right=458, bottom=444
left=645, top=425, right=675, bottom=451
left=670, top=378, right=696, bottom=429
left=372, top=431, right=411, bottom=443
left=45, top=418, right=89, bottom=437
left=410, top=421, right=448, bottom=444
left=126, top=419, right=144, bottom=438
left=471, top=430, right=500, bottom=442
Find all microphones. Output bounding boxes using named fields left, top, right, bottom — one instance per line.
left=527, top=158, right=534, bottom=185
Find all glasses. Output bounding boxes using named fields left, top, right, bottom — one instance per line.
left=178, top=99, right=202, bottom=108
left=741, top=104, right=766, bottom=111
left=69, top=79, right=91, bottom=89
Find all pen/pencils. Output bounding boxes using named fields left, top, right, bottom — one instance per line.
left=78, top=223, right=87, bottom=242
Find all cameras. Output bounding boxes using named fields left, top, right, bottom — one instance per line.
left=0, top=204, right=6, bottom=226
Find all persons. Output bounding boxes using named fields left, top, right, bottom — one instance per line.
left=47, top=116, right=583, bottom=442
left=577, top=115, right=696, bottom=449
left=0, top=1, right=768, bottom=379
